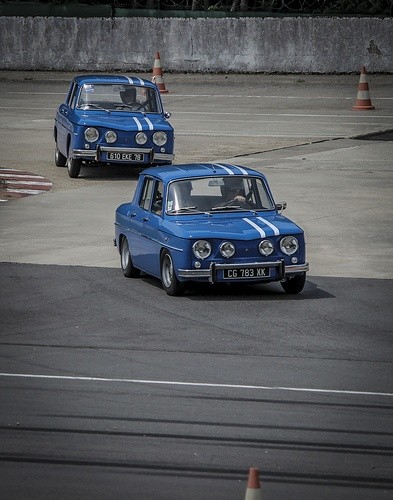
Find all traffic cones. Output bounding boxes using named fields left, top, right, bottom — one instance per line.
left=150, top=51, right=170, bottom=94
left=352, top=65, right=377, bottom=111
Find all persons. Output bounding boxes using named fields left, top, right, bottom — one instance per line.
left=210, top=178, right=257, bottom=208
left=116, top=87, right=150, bottom=113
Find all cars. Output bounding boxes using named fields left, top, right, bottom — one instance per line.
left=53, top=73, right=175, bottom=177
left=112, top=161, right=311, bottom=297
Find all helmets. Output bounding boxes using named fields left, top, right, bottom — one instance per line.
left=120, top=85, right=137, bottom=103
left=220, top=178, right=244, bottom=201
